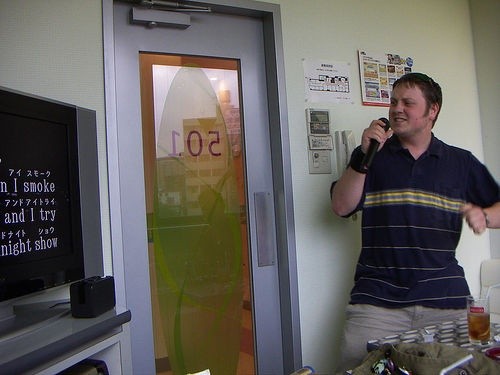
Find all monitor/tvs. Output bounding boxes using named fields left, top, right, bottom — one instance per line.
left=0, top=86, right=104, bottom=307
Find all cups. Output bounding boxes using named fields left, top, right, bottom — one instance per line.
left=467, top=296, right=491, bottom=344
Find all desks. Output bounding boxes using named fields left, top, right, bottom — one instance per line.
left=366, top=303, right=499, bottom=375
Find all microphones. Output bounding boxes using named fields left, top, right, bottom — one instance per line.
left=359, top=117, right=390, bottom=170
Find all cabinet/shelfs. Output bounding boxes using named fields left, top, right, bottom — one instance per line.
left=30, top=332, right=133, bottom=375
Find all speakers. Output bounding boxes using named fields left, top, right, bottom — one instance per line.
left=71, top=275, right=116, bottom=318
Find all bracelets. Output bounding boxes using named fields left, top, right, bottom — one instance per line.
left=479, top=209, right=490, bottom=230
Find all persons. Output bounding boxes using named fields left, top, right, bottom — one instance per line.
left=330, top=72, right=500, bottom=375
